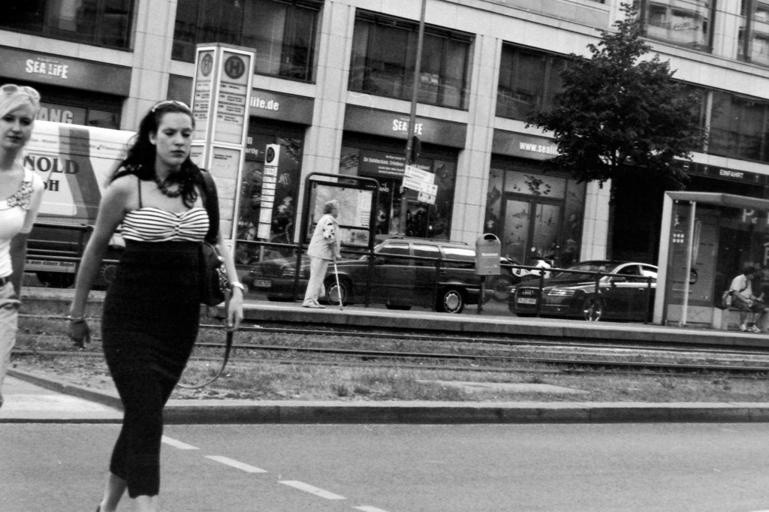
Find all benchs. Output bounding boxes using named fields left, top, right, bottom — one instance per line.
left=712, top=304, right=769, bottom=332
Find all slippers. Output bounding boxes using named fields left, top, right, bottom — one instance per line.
left=152, top=100, right=190, bottom=113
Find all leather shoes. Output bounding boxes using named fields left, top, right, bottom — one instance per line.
left=308, top=303, right=326, bottom=308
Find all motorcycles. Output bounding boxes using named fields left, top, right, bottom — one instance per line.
left=485, top=243, right=560, bottom=302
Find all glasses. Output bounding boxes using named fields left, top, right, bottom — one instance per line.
left=0, top=83, right=40, bottom=99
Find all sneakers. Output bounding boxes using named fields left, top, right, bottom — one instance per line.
left=739, top=324, right=761, bottom=333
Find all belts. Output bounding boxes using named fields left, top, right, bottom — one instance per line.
left=0, top=277, right=9, bottom=287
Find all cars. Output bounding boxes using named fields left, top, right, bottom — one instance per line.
left=242, top=234, right=495, bottom=313
left=508, top=260, right=658, bottom=322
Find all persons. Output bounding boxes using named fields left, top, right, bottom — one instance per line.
left=0, top=79, right=46, bottom=410
left=302, top=199, right=342, bottom=308
left=715, top=258, right=768, bottom=334
left=275, top=196, right=296, bottom=244
left=61, top=96, right=247, bottom=512
left=237, top=169, right=263, bottom=241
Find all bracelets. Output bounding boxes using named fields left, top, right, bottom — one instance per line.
left=229, top=281, right=245, bottom=292
left=65, top=314, right=85, bottom=324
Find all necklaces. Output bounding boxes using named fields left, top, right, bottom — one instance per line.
left=152, top=169, right=184, bottom=197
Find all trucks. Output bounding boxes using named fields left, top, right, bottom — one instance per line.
left=23, top=119, right=141, bottom=290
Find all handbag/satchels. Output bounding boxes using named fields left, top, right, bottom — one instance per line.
left=199, top=168, right=233, bottom=307
left=721, top=289, right=733, bottom=306
